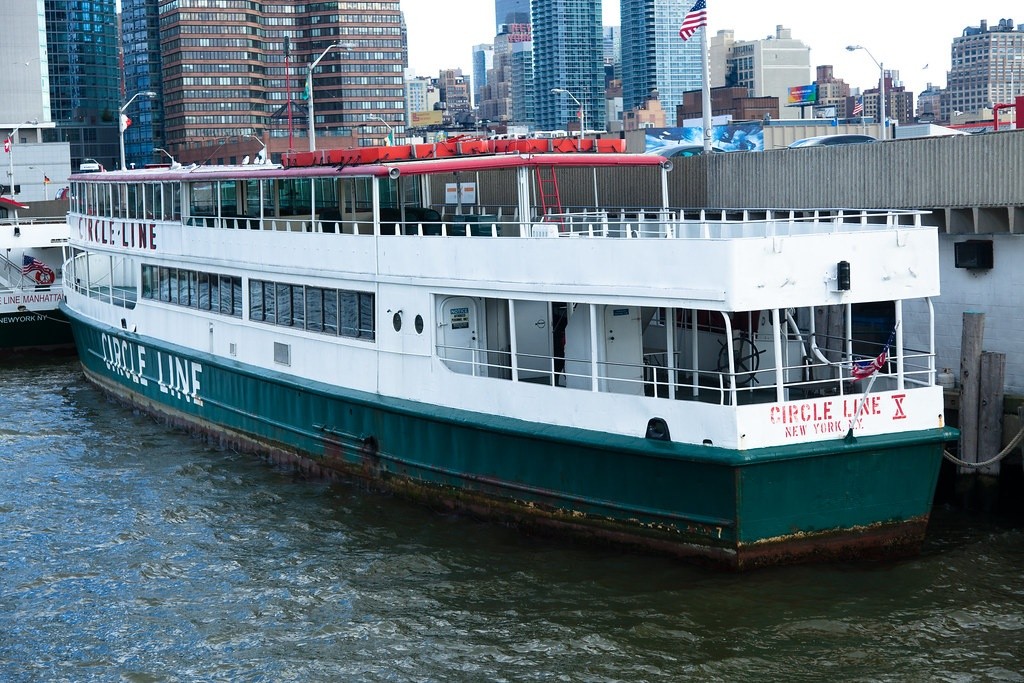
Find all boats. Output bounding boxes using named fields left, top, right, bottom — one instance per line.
left=59, top=137, right=959, bottom=582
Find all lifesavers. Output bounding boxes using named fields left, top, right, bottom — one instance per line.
left=36, top=268, right=55, bottom=285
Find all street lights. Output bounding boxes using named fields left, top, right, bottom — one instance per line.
left=118, top=92, right=156, bottom=169
left=7, top=121, right=38, bottom=214
left=29, top=166, right=48, bottom=201
left=243, top=134, right=267, bottom=163
left=153, top=148, right=174, bottom=166
left=84, top=159, right=100, bottom=166
left=306, top=43, right=357, bottom=151
left=846, top=44, right=890, bottom=139
left=366, top=117, right=395, bottom=146
left=551, top=88, right=584, bottom=139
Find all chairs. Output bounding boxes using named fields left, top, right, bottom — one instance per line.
left=445, top=214, right=505, bottom=238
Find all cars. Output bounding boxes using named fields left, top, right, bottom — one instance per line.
left=55, top=186, right=69, bottom=200
left=644, top=144, right=726, bottom=157
left=787, top=134, right=876, bottom=148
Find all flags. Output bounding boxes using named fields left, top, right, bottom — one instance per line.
left=679, top=0, right=708, bottom=41
left=22, top=255, right=49, bottom=275
left=301, top=71, right=310, bottom=100
left=850, top=329, right=896, bottom=382
left=853, top=96, right=863, bottom=114
